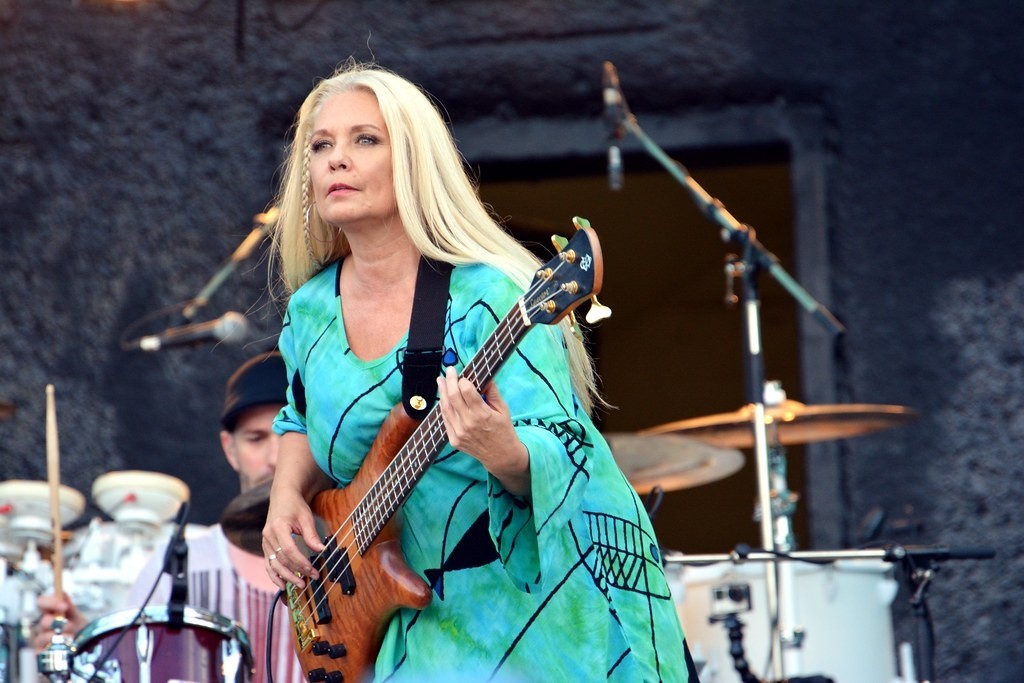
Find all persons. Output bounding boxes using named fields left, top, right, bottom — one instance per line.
left=23, top=350, right=307, bottom=682
left=257, top=67, right=691, bottom=682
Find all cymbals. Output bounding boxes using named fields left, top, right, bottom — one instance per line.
left=599, top=414, right=744, bottom=497
left=641, top=381, right=915, bottom=446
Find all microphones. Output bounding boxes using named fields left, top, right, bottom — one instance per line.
left=600, top=61, right=627, bottom=141
left=141, top=311, right=249, bottom=352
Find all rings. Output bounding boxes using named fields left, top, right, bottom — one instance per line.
left=267, top=554, right=277, bottom=564
left=275, top=548, right=283, bottom=557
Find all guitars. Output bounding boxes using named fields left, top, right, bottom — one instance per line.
left=286, top=216, right=612, bottom=683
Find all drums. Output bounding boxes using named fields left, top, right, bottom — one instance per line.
left=37, top=605, right=254, bottom=683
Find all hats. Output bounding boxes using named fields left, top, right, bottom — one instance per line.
left=219, top=351, right=291, bottom=428
